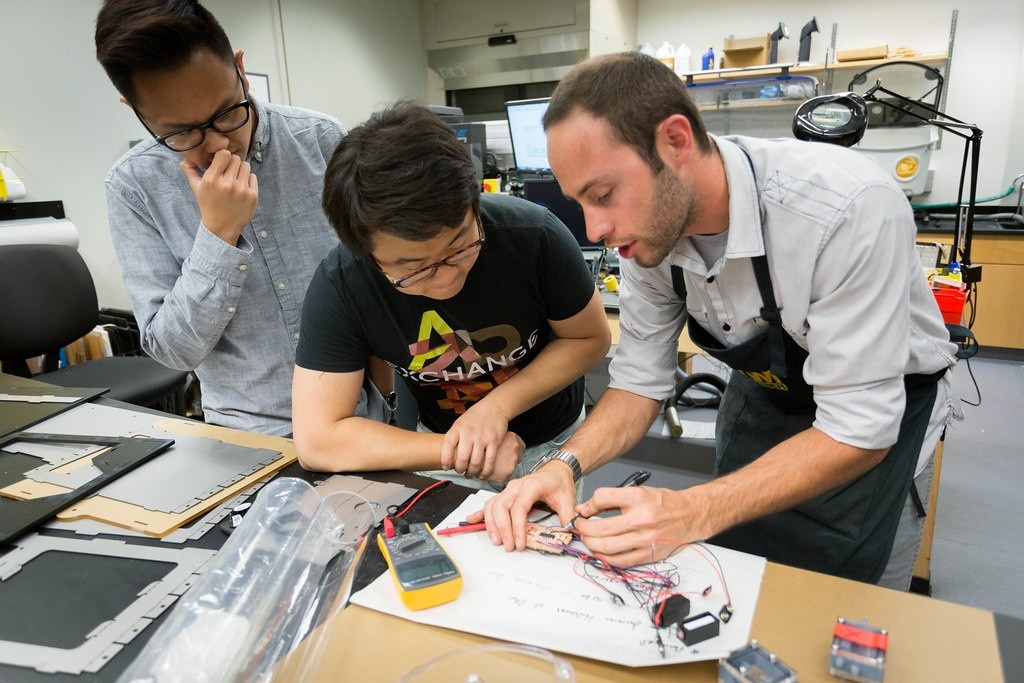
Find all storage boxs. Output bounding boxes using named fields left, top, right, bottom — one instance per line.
left=723, top=36, right=772, bottom=68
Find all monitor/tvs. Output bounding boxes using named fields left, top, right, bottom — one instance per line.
left=524, top=179, right=606, bottom=250
left=504, top=97, right=554, bottom=175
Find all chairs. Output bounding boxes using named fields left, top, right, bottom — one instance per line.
left=0, top=244, right=187, bottom=419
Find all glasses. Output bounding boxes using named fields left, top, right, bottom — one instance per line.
left=131, top=61, right=250, bottom=152
left=368, top=200, right=486, bottom=288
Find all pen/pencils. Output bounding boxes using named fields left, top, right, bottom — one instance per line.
left=562, top=470, right=652, bottom=531
left=436, top=524, right=487, bottom=537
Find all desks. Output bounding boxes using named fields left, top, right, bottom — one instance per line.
left=0, top=370, right=1004, bottom=683
left=602, top=310, right=966, bottom=595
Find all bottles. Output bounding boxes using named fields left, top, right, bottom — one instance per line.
left=640, top=41, right=655, bottom=59
left=655, top=41, right=674, bottom=72
left=675, top=42, right=690, bottom=74
left=702, top=46, right=715, bottom=70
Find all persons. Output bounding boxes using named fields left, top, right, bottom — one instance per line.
left=465, top=50, right=964, bottom=584
left=96, top=0, right=400, bottom=437
left=291, top=100, right=611, bottom=505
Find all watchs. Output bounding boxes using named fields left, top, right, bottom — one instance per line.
left=529, top=449, right=582, bottom=484
left=384, top=392, right=397, bottom=409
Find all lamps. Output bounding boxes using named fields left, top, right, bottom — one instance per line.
left=793, top=81, right=984, bottom=283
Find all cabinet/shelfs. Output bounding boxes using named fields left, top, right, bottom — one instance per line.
left=679, top=52, right=949, bottom=111
left=914, top=232, right=1024, bottom=363
